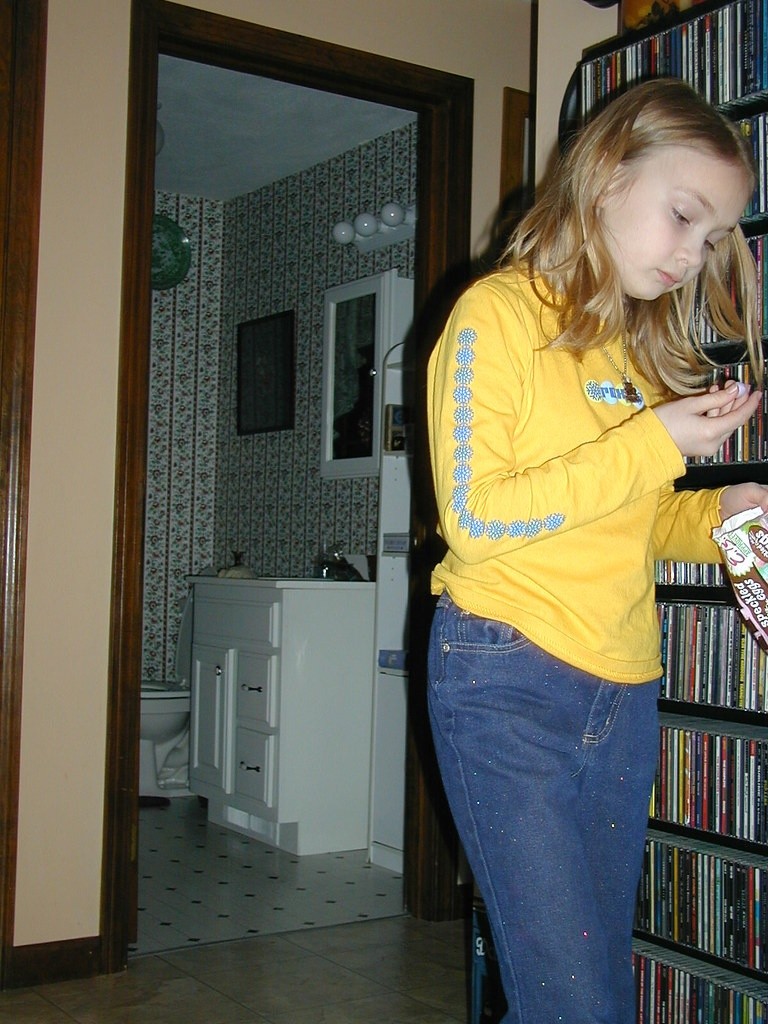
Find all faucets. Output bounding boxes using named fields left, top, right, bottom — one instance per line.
left=318, top=539, right=349, bottom=578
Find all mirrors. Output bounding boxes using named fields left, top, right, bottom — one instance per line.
left=235, top=306, right=302, bottom=436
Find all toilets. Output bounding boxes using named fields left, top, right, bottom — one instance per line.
left=138, top=567, right=219, bottom=799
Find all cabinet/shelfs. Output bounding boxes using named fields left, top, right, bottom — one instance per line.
left=364, top=342, right=408, bottom=873
left=321, top=265, right=415, bottom=478
left=184, top=575, right=376, bottom=856
left=567, top=0, right=768, bottom=1024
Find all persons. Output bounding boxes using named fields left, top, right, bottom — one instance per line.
left=427, top=79, right=768, bottom=1024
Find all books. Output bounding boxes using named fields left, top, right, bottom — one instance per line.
left=473, top=907, right=508, bottom=1024
left=632, top=562, right=768, bottom=1024
left=585, top=0, right=768, bottom=217
left=683, top=234, right=768, bottom=466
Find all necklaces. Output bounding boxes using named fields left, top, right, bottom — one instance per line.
left=603, top=331, right=640, bottom=402
